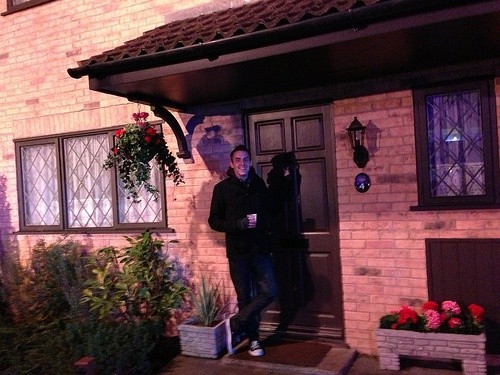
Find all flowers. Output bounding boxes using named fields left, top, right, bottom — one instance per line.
left=378, top=300, right=486, bottom=336
left=103, top=112, right=185, bottom=203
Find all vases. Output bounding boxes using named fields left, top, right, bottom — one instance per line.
left=376, top=326, right=488, bottom=375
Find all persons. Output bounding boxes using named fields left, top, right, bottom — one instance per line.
left=209, top=144, right=277, bottom=358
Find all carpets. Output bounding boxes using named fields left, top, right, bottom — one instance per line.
left=228, top=338, right=334, bottom=368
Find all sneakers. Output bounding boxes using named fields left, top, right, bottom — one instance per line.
left=226, top=318, right=240, bottom=355
left=248, top=341, right=264, bottom=356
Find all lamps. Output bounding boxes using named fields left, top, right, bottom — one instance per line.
left=345, top=116, right=370, bottom=168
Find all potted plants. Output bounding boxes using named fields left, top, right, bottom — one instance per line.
left=177, top=273, right=231, bottom=360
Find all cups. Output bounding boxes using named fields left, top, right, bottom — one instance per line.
left=247, top=214, right=257, bottom=228
